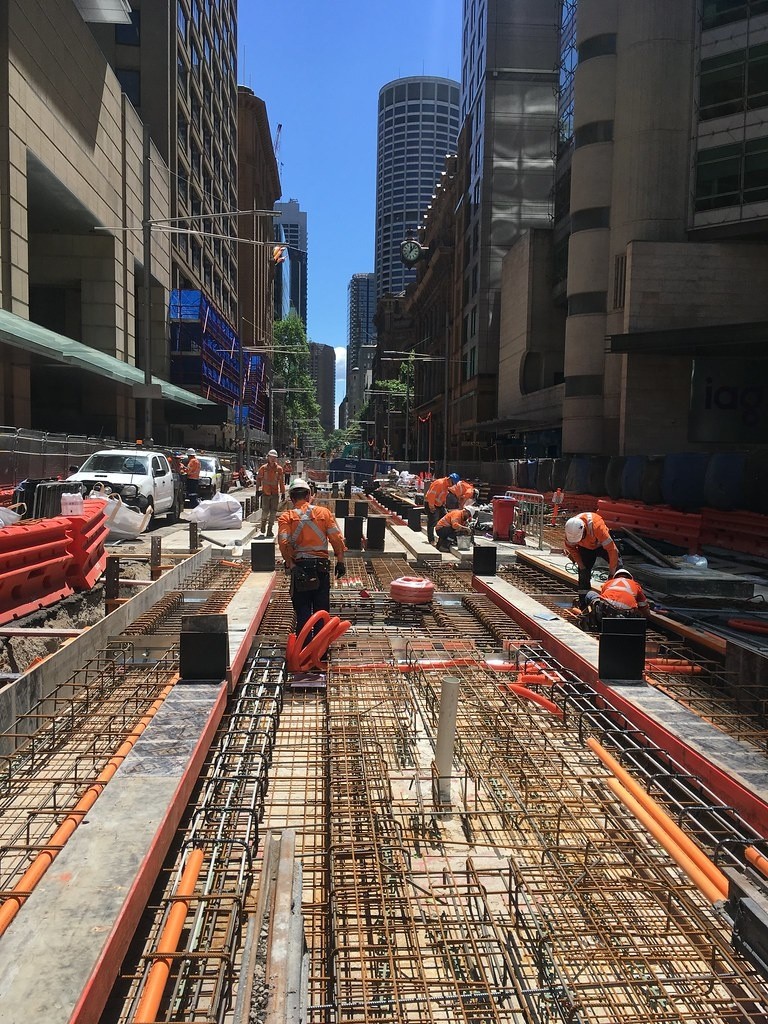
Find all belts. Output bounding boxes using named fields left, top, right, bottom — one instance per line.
left=603, top=600, right=635, bottom=613
left=296, top=558, right=328, bottom=561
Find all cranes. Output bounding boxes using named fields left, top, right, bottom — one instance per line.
left=274, top=123, right=284, bottom=158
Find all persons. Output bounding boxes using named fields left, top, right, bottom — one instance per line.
left=166, top=448, right=201, bottom=508
left=283, top=460, right=293, bottom=485
left=256, top=449, right=285, bottom=536
left=564, top=512, right=623, bottom=611
left=424, top=473, right=479, bottom=549
left=277, top=478, right=348, bottom=662
left=585, top=568, right=650, bottom=633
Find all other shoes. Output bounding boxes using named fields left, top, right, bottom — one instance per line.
left=430, top=541, right=437, bottom=545
left=439, top=546, right=448, bottom=551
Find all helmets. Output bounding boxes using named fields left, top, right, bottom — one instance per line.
left=613, top=569, right=633, bottom=579
left=464, top=506, right=475, bottom=518
left=268, top=450, right=278, bottom=457
left=290, top=478, right=310, bottom=490
left=475, top=489, right=479, bottom=499
left=565, top=518, right=584, bottom=542
left=450, top=473, right=460, bottom=484
left=187, top=448, right=196, bottom=455
left=286, top=460, right=290, bottom=463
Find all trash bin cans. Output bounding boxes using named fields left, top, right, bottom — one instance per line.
left=491, top=498, right=517, bottom=540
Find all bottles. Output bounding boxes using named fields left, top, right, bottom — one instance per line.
left=61, top=492, right=84, bottom=515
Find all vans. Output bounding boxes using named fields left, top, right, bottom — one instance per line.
left=180, top=452, right=225, bottom=500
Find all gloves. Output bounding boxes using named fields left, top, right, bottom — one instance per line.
left=430, top=506, right=436, bottom=513
left=256, top=491, right=264, bottom=496
left=335, top=562, right=346, bottom=579
left=580, top=568, right=590, bottom=579
left=461, top=526, right=471, bottom=532
left=290, top=567, right=299, bottom=574
left=281, top=494, right=285, bottom=502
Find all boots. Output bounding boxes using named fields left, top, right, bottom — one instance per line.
left=267, top=526, right=274, bottom=536
left=261, top=521, right=266, bottom=533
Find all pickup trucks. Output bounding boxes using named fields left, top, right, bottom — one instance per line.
left=64, top=449, right=184, bottom=533
